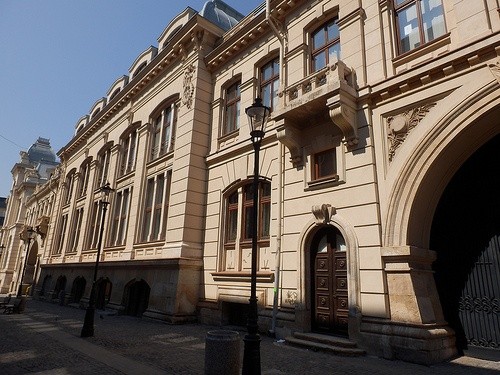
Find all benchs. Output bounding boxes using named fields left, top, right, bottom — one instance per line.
left=0, top=297, right=10, bottom=309
left=3, top=299, right=22, bottom=314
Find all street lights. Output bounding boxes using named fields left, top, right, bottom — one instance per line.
left=0, top=244, right=6, bottom=259
left=240, top=96, right=272, bottom=375
left=79, top=182, right=115, bottom=337
left=13, top=227, right=36, bottom=312
